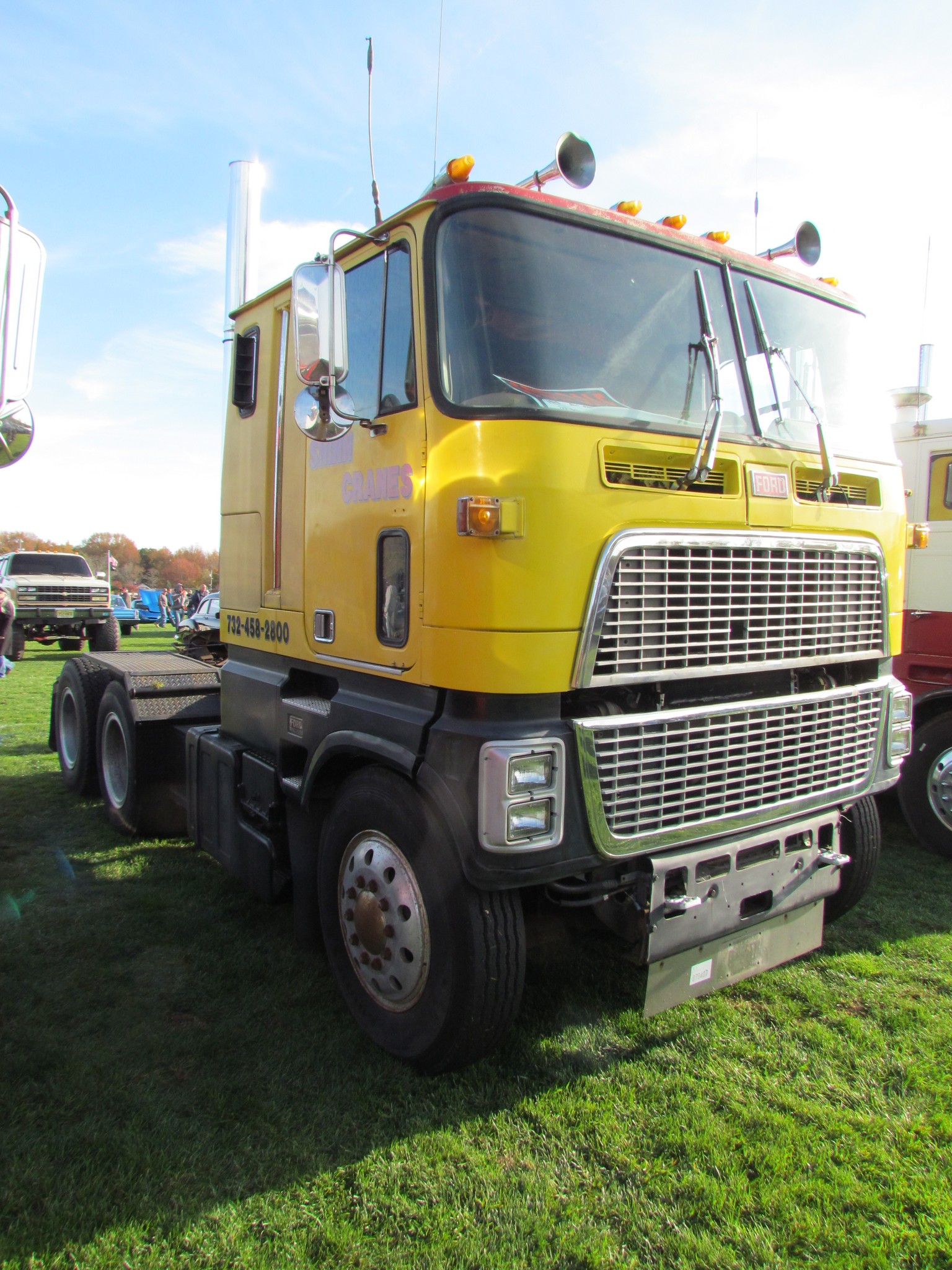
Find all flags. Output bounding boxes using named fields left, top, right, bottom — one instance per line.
left=109, top=552, right=118, bottom=571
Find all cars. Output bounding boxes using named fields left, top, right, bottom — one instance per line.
left=175, top=590, right=221, bottom=643
left=110, top=594, right=141, bottom=636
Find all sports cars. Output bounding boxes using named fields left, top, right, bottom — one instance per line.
left=131, top=588, right=177, bottom=623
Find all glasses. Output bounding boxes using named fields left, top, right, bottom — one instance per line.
left=163, top=591, right=167, bottom=592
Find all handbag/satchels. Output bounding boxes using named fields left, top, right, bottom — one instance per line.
left=170, top=599, right=183, bottom=610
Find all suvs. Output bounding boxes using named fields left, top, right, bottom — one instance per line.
left=0, top=549, right=121, bottom=662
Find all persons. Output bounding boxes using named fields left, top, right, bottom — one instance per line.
left=0, top=586, right=15, bottom=677
left=115, top=583, right=210, bottom=635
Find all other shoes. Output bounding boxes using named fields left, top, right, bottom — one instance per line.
left=6, top=661, right=15, bottom=674
left=154, top=622, right=160, bottom=628
left=173, top=624, right=176, bottom=626
left=136, top=624, right=139, bottom=630
left=162, top=626, right=169, bottom=628
left=0, top=673, right=6, bottom=677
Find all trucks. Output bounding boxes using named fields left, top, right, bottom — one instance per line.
left=49, top=148, right=931, bottom=1079
left=892, top=393, right=951, bottom=860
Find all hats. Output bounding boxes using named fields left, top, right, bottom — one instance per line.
left=205, top=590, right=210, bottom=593
left=120, top=587, right=127, bottom=590
left=0, top=586, right=10, bottom=595
